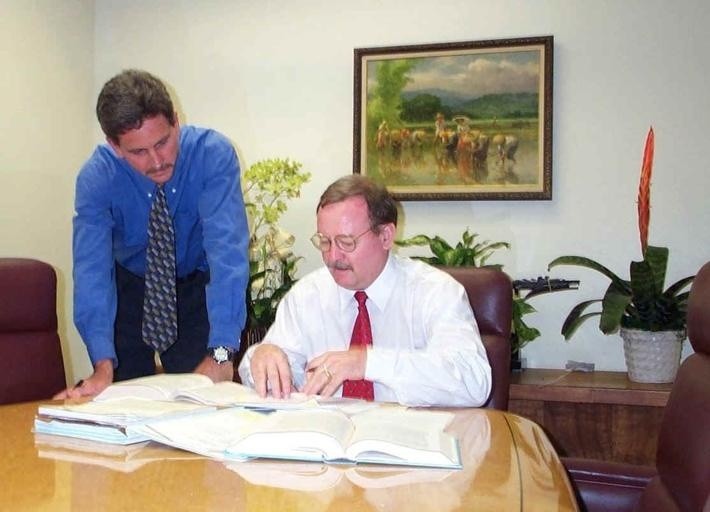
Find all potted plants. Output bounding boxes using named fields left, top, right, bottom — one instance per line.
left=548, top=126, right=697, bottom=385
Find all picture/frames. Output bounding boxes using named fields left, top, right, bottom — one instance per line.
left=352, top=35, right=553, bottom=201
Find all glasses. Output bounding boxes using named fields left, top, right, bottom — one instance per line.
left=310, top=226, right=373, bottom=252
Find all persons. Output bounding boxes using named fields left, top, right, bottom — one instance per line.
left=49, top=68, right=254, bottom=404
left=373, top=111, right=521, bottom=178
left=237, top=174, right=493, bottom=408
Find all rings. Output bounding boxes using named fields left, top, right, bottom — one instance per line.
left=323, top=367, right=333, bottom=381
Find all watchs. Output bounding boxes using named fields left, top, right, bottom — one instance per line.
left=204, top=345, right=239, bottom=365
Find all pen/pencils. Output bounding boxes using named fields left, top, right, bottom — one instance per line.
left=72, top=379, right=84, bottom=392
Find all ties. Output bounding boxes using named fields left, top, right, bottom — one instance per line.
left=342, top=291, right=374, bottom=401
left=141, top=182, right=179, bottom=356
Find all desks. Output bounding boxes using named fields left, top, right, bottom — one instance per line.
left=0, top=397, right=580, bottom=512
left=509, top=368, right=672, bottom=467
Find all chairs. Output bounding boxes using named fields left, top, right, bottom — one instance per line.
left=0, top=258, right=66, bottom=405
left=433, top=264, right=513, bottom=412
left=559, top=261, right=710, bottom=512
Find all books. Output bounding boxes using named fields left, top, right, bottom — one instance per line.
left=226, top=409, right=463, bottom=467
left=94, top=373, right=251, bottom=407
left=34, top=403, right=268, bottom=460
